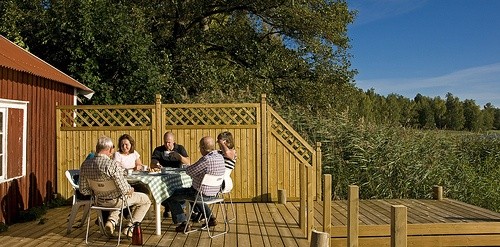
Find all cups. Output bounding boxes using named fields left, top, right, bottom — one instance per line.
left=161, top=167, right=169, bottom=174
left=141, top=164, right=147, bottom=171
left=164, top=150, right=170, bottom=157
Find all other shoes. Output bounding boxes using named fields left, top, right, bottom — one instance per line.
left=95, top=219, right=107, bottom=226
left=122, top=226, right=134, bottom=237
left=202, top=218, right=218, bottom=228
left=177, top=221, right=191, bottom=232
left=192, top=212, right=201, bottom=223
left=163, top=211, right=172, bottom=218
left=105, top=221, right=116, bottom=237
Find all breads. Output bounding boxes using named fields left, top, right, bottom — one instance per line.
left=148, top=168, right=160, bottom=172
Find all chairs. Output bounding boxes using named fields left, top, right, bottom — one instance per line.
left=65, top=169, right=236, bottom=246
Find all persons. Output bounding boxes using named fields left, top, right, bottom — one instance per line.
left=82, top=136, right=151, bottom=239
left=190, top=131, right=238, bottom=226
left=167, top=136, right=225, bottom=231
left=150, top=131, right=189, bottom=218
left=115, top=134, right=142, bottom=220
left=77, top=145, right=115, bottom=226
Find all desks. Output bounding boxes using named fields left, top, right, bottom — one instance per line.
left=123, top=172, right=194, bottom=235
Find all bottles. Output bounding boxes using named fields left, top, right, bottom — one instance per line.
left=132, top=222, right=143, bottom=246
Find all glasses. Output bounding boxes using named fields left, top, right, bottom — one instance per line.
left=112, top=145, right=115, bottom=149
